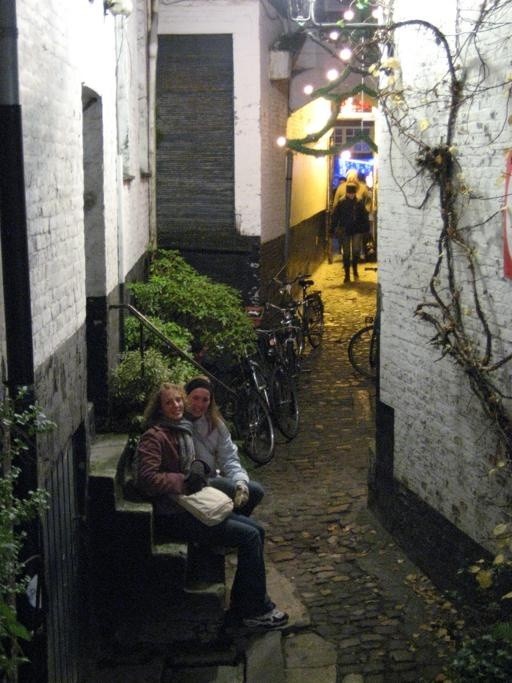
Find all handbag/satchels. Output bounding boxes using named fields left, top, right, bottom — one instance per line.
left=173, top=460, right=233, bottom=527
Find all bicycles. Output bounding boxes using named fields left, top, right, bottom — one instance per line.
left=234, top=301, right=309, bottom=466
left=271, top=274, right=323, bottom=347
left=348, top=268, right=380, bottom=379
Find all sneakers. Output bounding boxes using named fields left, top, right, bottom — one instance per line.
left=244, top=608, right=288, bottom=628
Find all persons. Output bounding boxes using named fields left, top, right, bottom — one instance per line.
left=132, top=384, right=289, bottom=627
left=333, top=169, right=366, bottom=261
left=183, top=377, right=264, bottom=518
left=331, top=185, right=369, bottom=284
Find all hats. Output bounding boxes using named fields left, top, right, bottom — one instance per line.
left=346, top=184, right=356, bottom=193
